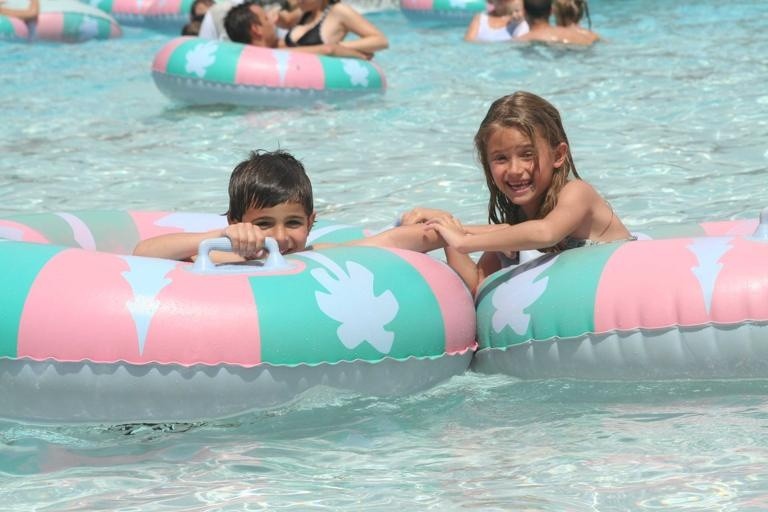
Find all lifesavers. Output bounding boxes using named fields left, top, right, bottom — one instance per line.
left=1, top=0, right=232, bottom=44
left=0, top=209, right=478, bottom=427
left=400, top=1, right=488, bottom=17
left=150, top=35, right=385, bottom=108
left=470, top=208, right=768, bottom=384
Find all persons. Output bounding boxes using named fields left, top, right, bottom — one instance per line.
left=132, top=149, right=516, bottom=265
left=180, top=0, right=389, bottom=61
left=463, top=0, right=598, bottom=49
left=0, top=1, right=39, bottom=22
left=400, top=91, right=637, bottom=299
